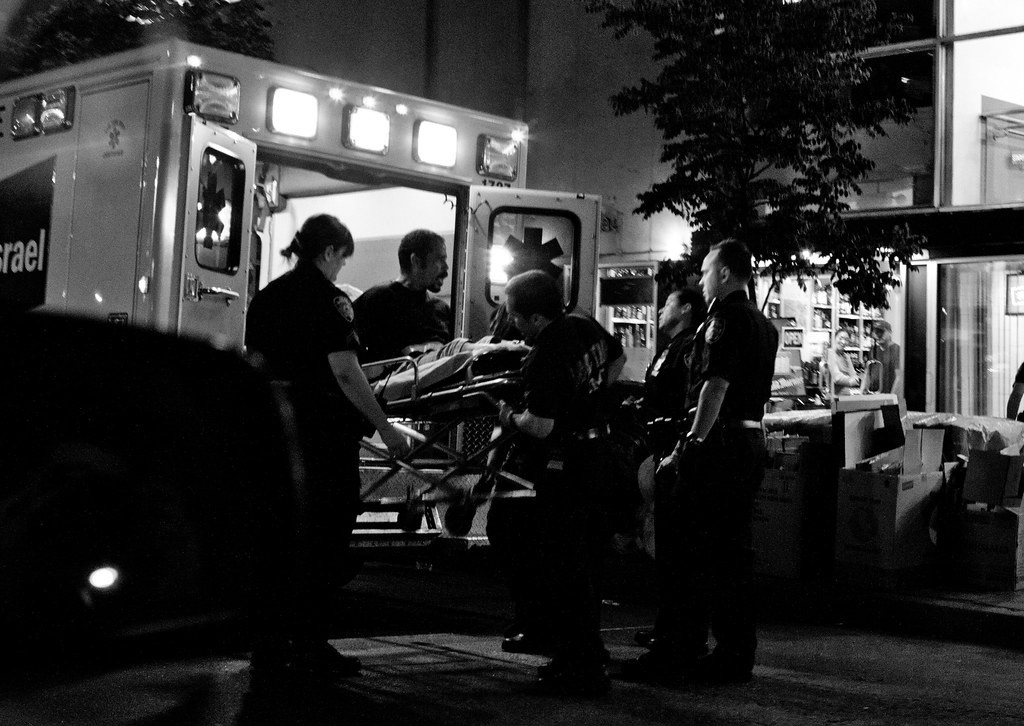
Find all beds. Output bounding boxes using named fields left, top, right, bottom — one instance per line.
left=359, top=346, right=533, bottom=537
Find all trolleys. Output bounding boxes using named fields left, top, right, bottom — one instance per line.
left=355, top=359, right=527, bottom=537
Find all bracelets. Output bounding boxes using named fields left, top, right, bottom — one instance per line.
left=687, top=430, right=703, bottom=444
left=506, top=410, right=517, bottom=428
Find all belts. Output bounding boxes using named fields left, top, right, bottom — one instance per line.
left=562, top=423, right=612, bottom=442
left=724, top=418, right=767, bottom=431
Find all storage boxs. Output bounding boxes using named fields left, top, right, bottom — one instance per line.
left=834, top=406, right=944, bottom=570
left=950, top=449, right=1024, bottom=592
left=755, top=469, right=803, bottom=577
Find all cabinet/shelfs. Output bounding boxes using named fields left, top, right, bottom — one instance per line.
left=598, top=262, right=889, bottom=373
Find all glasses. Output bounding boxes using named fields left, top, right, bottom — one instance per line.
left=424, top=253, right=446, bottom=266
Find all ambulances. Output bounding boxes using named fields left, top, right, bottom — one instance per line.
left=1, top=39, right=602, bottom=546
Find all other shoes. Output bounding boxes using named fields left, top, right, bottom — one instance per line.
left=691, top=643, right=752, bottom=686
left=501, top=630, right=539, bottom=655
left=248, top=638, right=291, bottom=671
left=531, top=658, right=614, bottom=700
left=609, top=650, right=687, bottom=691
left=282, top=640, right=363, bottom=676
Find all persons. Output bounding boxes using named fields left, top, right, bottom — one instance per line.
left=246, top=215, right=778, bottom=698
left=819, top=320, right=1024, bottom=423
